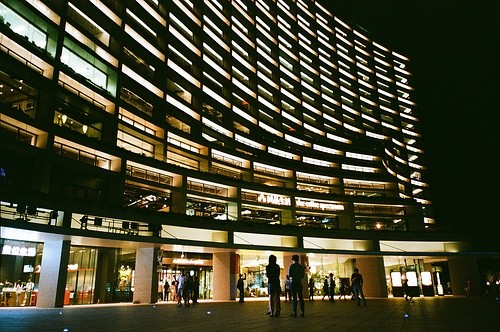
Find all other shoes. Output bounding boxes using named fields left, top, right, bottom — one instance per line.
left=289, top=312, right=297, bottom=317
left=298, top=312, right=304, bottom=316
left=269, top=312, right=279, bottom=317
left=265, top=311, right=271, bottom=315
left=176, top=303, right=183, bottom=307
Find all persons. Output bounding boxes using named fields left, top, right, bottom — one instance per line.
left=12, top=279, right=20, bottom=292
left=172, top=270, right=200, bottom=307
left=3, top=278, right=13, bottom=298
left=237, top=272, right=246, bottom=303
left=400, top=274, right=414, bottom=301
left=321, top=273, right=348, bottom=303
left=118, top=279, right=127, bottom=291
left=282, top=274, right=293, bottom=302
left=350, top=268, right=366, bottom=305
left=265, top=254, right=281, bottom=317
left=308, top=277, right=314, bottom=301
left=288, top=254, right=306, bottom=317
left=163, top=282, right=170, bottom=301
left=19, top=278, right=34, bottom=307
left=443, top=278, right=500, bottom=304
